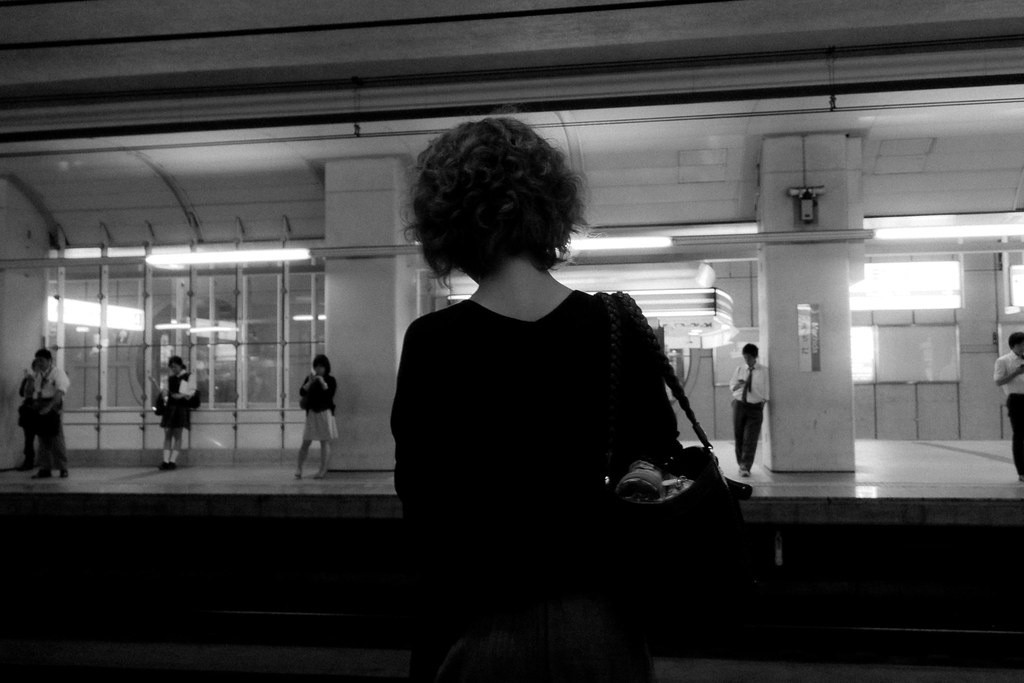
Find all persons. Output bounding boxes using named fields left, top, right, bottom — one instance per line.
left=294, top=354, right=339, bottom=479
left=729, top=344, right=770, bottom=478
left=157, top=356, right=197, bottom=470
left=17, top=359, right=40, bottom=470
left=30, top=349, right=71, bottom=479
left=994, top=332, right=1024, bottom=481
left=389, top=116, right=683, bottom=683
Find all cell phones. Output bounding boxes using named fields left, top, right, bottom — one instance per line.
left=738, top=379, right=744, bottom=382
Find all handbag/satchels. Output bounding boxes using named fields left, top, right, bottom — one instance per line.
left=596, top=291, right=747, bottom=630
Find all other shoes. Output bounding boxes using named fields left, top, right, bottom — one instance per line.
left=313, top=470, right=327, bottom=480
left=32, top=469, right=52, bottom=480
left=294, top=469, right=302, bottom=478
left=60, top=468, right=68, bottom=478
left=159, top=461, right=177, bottom=470
left=740, top=468, right=749, bottom=477
